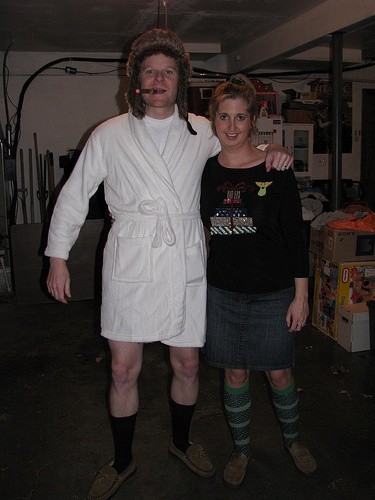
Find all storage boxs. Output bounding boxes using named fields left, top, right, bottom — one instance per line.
left=309, top=224, right=375, bottom=353
left=282, top=89, right=322, bottom=123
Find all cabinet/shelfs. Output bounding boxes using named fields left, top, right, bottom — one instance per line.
left=282, top=122, right=313, bottom=180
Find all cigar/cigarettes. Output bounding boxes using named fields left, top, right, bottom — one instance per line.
left=135, top=89, right=157, bottom=94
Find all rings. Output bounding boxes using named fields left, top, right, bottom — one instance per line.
left=282, top=164, right=287, bottom=167
left=301, top=322, right=306, bottom=326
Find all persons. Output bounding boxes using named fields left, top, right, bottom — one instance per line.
left=44, top=31, right=315, bottom=500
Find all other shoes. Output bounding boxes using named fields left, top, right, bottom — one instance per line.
left=285, top=443, right=318, bottom=477
left=169, top=434, right=215, bottom=478
left=222, top=447, right=250, bottom=488
left=87, top=455, right=135, bottom=500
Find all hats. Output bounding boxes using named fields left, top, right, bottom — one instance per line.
left=127, top=29, right=197, bottom=136
